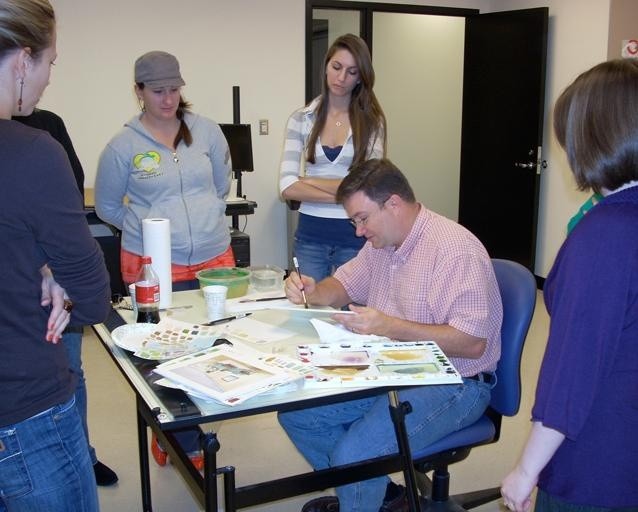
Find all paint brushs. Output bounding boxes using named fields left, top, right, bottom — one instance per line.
left=292, top=250, right=309, bottom=309
left=201, top=310, right=252, bottom=328
left=239, top=296, right=291, bottom=304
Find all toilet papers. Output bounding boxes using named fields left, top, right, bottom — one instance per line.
left=143, top=218, right=171, bottom=310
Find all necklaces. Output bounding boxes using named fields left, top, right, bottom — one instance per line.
left=325, top=105, right=351, bottom=128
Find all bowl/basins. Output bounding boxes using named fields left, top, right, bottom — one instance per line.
left=194, top=267, right=252, bottom=298
left=247, top=262, right=286, bottom=291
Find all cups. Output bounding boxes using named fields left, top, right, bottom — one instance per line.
left=128, top=283, right=135, bottom=307
left=203, top=285, right=228, bottom=321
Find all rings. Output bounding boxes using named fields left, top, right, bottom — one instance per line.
left=63, top=298, right=75, bottom=313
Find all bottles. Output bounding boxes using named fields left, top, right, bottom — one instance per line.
left=136, top=254, right=160, bottom=326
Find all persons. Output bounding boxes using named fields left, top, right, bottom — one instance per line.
left=278, top=33, right=388, bottom=310
left=277, top=158, right=504, bottom=512
left=94, top=49, right=236, bottom=471
left=0, top=0, right=111, bottom=512
left=499, top=56, right=638, bottom=512
left=11, top=108, right=119, bottom=487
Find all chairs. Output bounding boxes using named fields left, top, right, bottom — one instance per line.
left=387, top=259, right=536, bottom=511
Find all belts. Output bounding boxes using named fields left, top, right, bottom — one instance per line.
left=465, top=371, right=497, bottom=385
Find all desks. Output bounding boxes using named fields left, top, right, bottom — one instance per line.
left=85, top=197, right=257, bottom=233
left=91, top=275, right=449, bottom=512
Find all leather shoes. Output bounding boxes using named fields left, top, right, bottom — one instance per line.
left=188, top=454, right=204, bottom=469
left=91, top=460, right=119, bottom=487
left=150, top=427, right=169, bottom=467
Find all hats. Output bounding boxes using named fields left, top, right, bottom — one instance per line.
left=134, top=50, right=185, bottom=90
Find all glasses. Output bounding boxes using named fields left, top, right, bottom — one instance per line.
left=349, top=202, right=383, bottom=227
